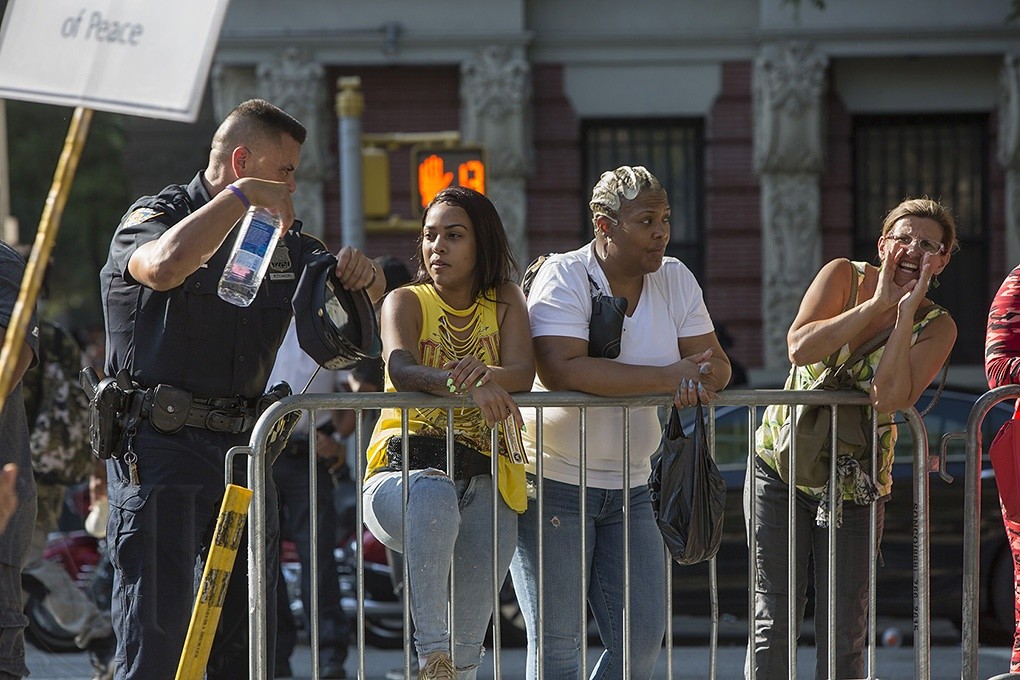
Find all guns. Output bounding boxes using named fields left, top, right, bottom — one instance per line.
left=79, top=368, right=116, bottom=459
left=109, top=369, right=153, bottom=459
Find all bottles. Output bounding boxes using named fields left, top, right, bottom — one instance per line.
left=217, top=205, right=283, bottom=307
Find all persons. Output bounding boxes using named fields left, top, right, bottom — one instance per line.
left=373, top=255, right=412, bottom=292
left=743, top=197, right=957, bottom=680
left=98, top=100, right=387, bottom=680
left=363, top=187, right=537, bottom=680
left=0, top=242, right=41, bottom=680
left=984, top=268, right=1020, bottom=672
left=510, top=166, right=732, bottom=680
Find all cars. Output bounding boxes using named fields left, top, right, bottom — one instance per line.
left=21, top=379, right=1017, bottom=653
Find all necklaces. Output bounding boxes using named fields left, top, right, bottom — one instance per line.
left=442, top=316, right=457, bottom=359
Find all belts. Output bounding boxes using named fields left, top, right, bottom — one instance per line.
left=141, top=395, right=257, bottom=434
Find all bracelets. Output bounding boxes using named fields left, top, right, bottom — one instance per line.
left=226, top=184, right=249, bottom=208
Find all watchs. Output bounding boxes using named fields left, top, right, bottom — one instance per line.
left=365, top=261, right=378, bottom=289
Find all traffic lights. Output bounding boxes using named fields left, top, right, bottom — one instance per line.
left=409, top=144, right=487, bottom=216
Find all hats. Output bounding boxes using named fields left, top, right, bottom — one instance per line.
left=292, top=255, right=383, bottom=371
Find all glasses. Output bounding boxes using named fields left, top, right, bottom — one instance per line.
left=886, top=233, right=946, bottom=255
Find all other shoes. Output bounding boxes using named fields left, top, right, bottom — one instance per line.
left=274, top=659, right=293, bottom=678
left=317, top=664, right=348, bottom=680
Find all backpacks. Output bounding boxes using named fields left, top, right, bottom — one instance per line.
left=774, top=263, right=951, bottom=487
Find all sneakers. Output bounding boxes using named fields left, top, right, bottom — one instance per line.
left=418, top=652, right=458, bottom=680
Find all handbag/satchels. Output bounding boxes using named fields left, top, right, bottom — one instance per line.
left=523, top=252, right=628, bottom=358
left=648, top=396, right=726, bottom=566
left=388, top=435, right=490, bottom=479
